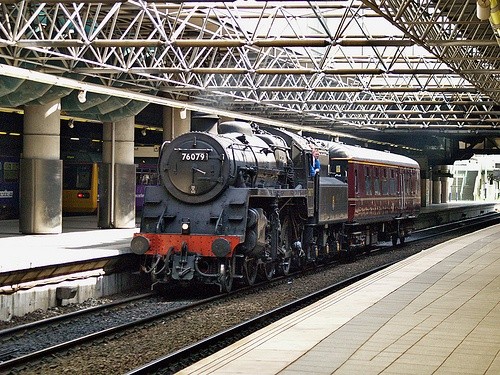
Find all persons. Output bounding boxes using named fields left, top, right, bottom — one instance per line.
left=310, top=150, right=320, bottom=177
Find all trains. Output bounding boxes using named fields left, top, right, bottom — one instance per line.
left=130, top=121, right=421, bottom=293
